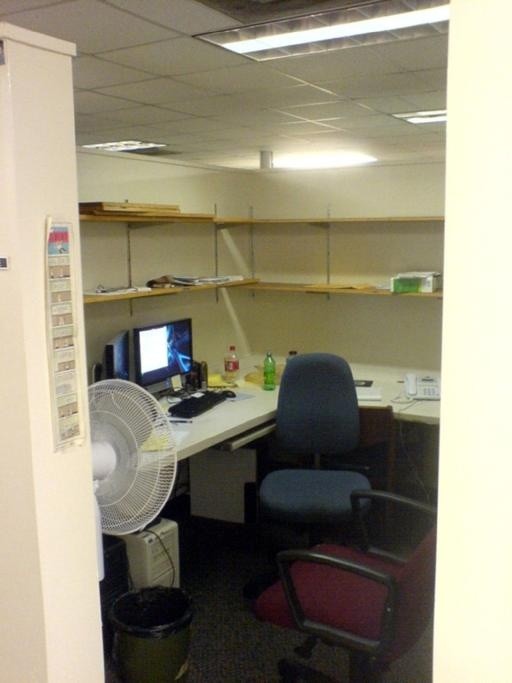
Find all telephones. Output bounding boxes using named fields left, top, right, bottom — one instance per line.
left=405, top=371, right=441, bottom=400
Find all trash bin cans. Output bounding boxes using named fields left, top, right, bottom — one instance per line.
left=108, top=587, right=195, bottom=682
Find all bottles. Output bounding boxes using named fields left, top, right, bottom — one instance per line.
left=224, top=345, right=239, bottom=385
left=263, top=351, right=277, bottom=391
left=286, top=350, right=296, bottom=362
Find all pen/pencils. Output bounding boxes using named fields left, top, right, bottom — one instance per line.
left=169, top=421, right=193, bottom=423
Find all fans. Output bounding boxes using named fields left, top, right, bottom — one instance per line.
left=85, top=378, right=180, bottom=582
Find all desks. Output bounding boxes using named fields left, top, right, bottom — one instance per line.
left=92, top=356, right=439, bottom=521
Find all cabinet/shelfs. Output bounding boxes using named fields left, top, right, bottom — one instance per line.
left=250, top=218, right=444, bottom=298
left=79, top=201, right=262, bottom=305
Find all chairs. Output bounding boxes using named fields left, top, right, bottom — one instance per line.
left=252, top=487, right=434, bottom=682
left=236, top=352, right=378, bottom=544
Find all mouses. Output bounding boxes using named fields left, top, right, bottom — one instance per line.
left=223, top=390, right=236, bottom=398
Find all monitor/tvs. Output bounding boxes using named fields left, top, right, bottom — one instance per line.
left=133, top=318, right=193, bottom=399
left=93, top=330, right=129, bottom=382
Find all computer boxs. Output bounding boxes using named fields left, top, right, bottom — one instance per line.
left=111, top=516, right=181, bottom=593
left=99, top=533, right=127, bottom=657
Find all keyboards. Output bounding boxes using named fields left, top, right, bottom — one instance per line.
left=168, top=390, right=226, bottom=419
